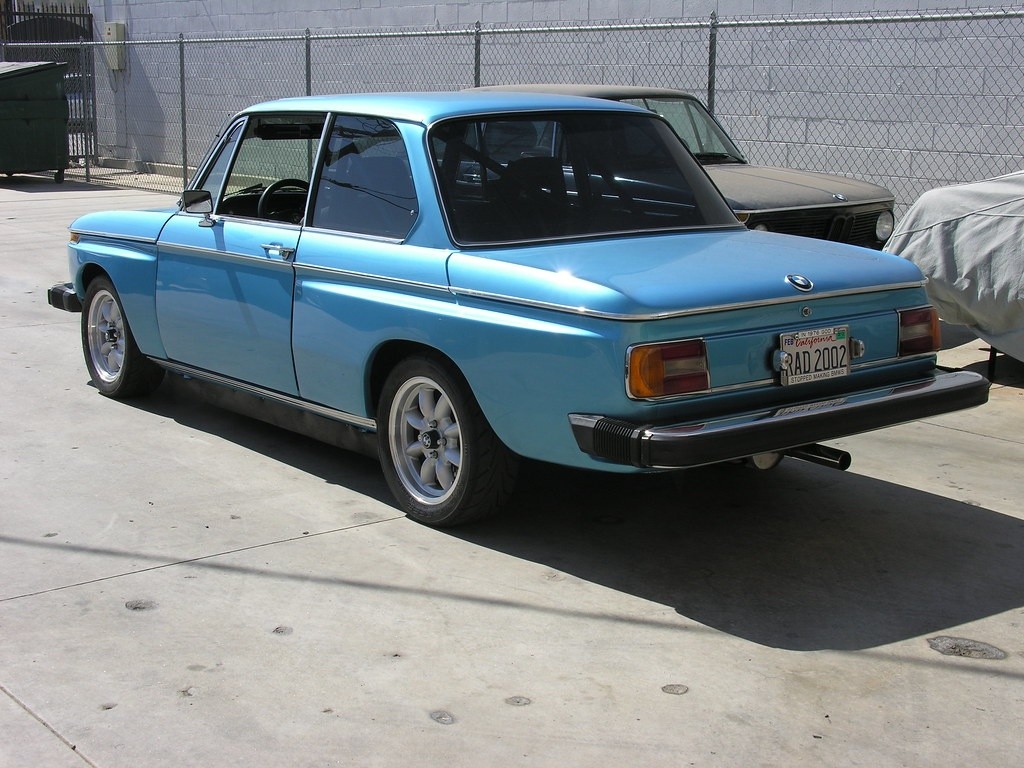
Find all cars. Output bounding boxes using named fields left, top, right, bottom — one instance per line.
left=46, top=89, right=994, bottom=530
left=399, top=84, right=895, bottom=253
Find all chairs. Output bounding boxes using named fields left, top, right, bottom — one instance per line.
left=494, top=156, right=568, bottom=229
left=327, top=157, right=414, bottom=233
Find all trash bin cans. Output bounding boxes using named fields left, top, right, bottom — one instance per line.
left=0, top=62, right=72, bottom=184
